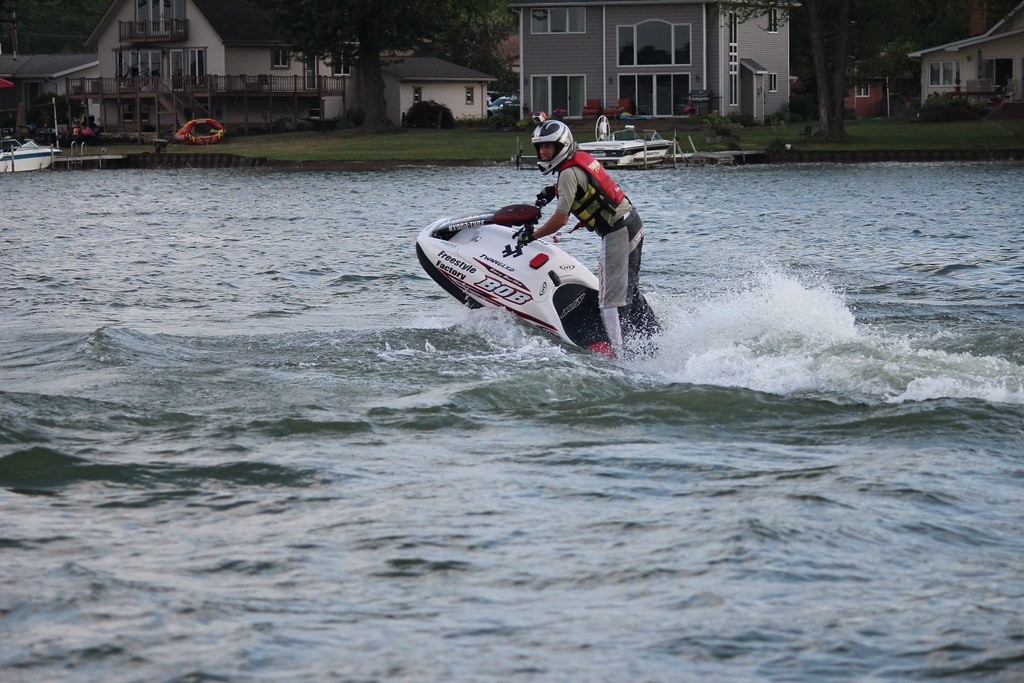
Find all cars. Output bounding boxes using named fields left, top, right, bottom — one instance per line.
left=487, top=97, right=519, bottom=117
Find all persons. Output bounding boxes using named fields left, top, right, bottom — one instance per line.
left=517, top=120, right=645, bottom=362
left=73, top=122, right=93, bottom=146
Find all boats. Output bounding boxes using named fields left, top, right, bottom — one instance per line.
left=0, top=137, right=62, bottom=173
left=619, top=112, right=648, bottom=120
left=579, top=126, right=670, bottom=171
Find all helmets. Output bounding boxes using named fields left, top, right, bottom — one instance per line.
left=532, top=120, right=574, bottom=175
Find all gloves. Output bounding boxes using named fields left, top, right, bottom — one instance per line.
left=536, top=186, right=556, bottom=204
left=516, top=231, right=536, bottom=246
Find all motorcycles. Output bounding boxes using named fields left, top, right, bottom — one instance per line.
left=414, top=184, right=664, bottom=365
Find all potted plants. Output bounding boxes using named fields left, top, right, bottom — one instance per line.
left=954, top=79, right=961, bottom=92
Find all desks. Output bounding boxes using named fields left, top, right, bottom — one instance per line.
left=947, top=91, right=992, bottom=98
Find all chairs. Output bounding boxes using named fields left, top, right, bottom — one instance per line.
left=799, top=126, right=813, bottom=144
left=966, top=79, right=1019, bottom=103
left=582, top=99, right=602, bottom=120
left=602, top=98, right=631, bottom=120
